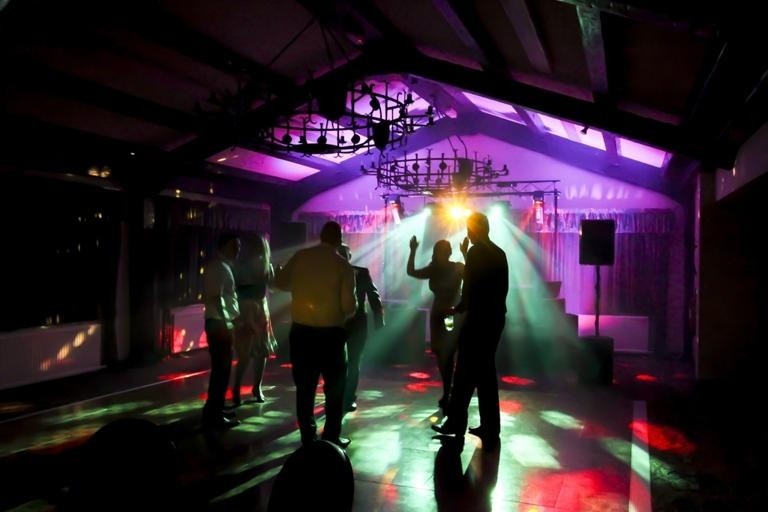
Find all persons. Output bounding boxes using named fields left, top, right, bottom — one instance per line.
left=432, top=435, right=499, bottom=512
left=407, top=234, right=469, bottom=417
left=200, top=220, right=386, bottom=451
left=430, top=213, right=508, bottom=437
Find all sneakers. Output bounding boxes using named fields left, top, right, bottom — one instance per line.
left=231, top=387, right=242, bottom=404
left=344, top=401, right=357, bottom=411
left=251, top=387, right=265, bottom=403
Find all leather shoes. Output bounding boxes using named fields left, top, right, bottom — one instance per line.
left=467, top=425, right=499, bottom=440
left=220, top=408, right=237, bottom=417
left=202, top=416, right=240, bottom=428
left=431, top=421, right=467, bottom=438
left=336, top=436, right=351, bottom=448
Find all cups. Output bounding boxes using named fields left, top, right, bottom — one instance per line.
left=444, top=315, right=454, bottom=332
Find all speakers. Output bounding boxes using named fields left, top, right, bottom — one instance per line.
left=268, top=220, right=307, bottom=261
left=579, top=219, right=616, bottom=265
left=577, top=336, right=613, bottom=386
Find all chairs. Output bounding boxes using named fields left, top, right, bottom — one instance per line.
left=266, top=440, right=354, bottom=512
left=55, top=416, right=177, bottom=511
